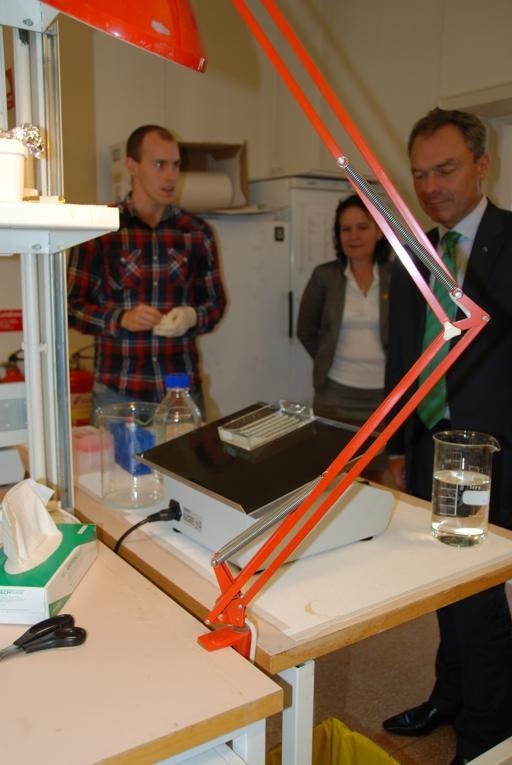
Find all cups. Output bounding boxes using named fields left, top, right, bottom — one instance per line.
left=431, top=431, right=500, bottom=553
left=94, top=402, right=171, bottom=512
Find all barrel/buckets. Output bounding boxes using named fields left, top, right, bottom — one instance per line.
left=1, top=139, right=29, bottom=204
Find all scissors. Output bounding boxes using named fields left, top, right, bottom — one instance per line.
left=0, top=614, right=86, bottom=662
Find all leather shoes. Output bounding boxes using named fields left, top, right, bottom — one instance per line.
left=382, top=699, right=453, bottom=737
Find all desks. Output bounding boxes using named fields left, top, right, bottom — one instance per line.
left=0, top=482, right=288, bottom=762
left=62, top=411, right=511, bottom=760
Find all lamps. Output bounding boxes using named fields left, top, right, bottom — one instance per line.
left=42, top=0, right=493, bottom=660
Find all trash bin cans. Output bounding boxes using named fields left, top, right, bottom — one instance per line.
left=266, top=715, right=402, bottom=765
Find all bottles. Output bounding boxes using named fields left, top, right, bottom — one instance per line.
left=153, top=374, right=201, bottom=445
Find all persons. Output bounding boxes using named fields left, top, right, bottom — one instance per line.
left=63, top=122, right=227, bottom=432
left=293, top=191, right=409, bottom=490
left=381, top=114, right=512, bottom=765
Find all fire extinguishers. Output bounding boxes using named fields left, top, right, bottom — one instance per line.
left=0, top=349, right=25, bottom=383
left=70, top=344, right=98, bottom=427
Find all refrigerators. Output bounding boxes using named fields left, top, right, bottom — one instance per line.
left=194, top=215, right=292, bottom=424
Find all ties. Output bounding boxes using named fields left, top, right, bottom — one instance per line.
left=416, top=232, right=462, bottom=430
